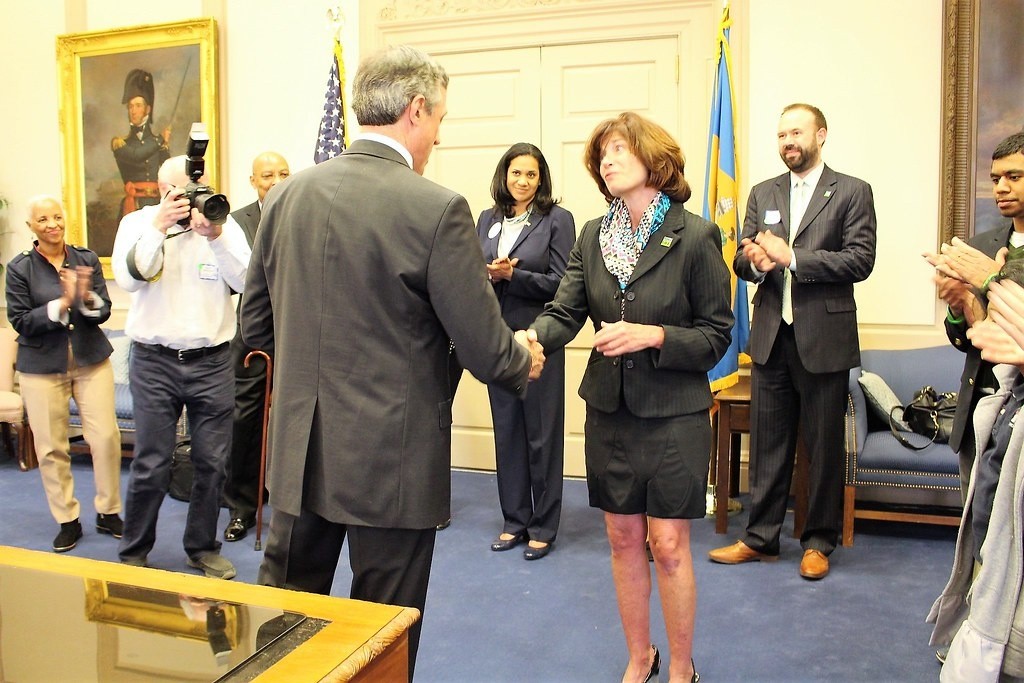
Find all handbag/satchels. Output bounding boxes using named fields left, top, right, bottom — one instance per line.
left=168, top=439, right=233, bottom=508
left=887, top=384, right=958, bottom=451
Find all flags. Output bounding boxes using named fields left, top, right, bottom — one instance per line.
left=313, top=28, right=349, bottom=164
left=699, top=1, right=751, bottom=388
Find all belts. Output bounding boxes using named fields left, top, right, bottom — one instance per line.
left=132, top=340, right=231, bottom=361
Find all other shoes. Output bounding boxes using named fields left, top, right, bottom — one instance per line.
left=96, top=513, right=124, bottom=539
left=646, top=541, right=654, bottom=561
left=689, top=657, right=699, bottom=683
left=643, top=644, right=661, bottom=683
left=120, top=558, right=149, bottom=568
left=52, top=517, right=83, bottom=552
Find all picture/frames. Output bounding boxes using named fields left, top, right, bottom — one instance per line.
left=53, top=16, right=220, bottom=280
left=84, top=577, right=242, bottom=650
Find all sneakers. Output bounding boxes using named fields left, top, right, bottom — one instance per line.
left=186, top=554, right=236, bottom=580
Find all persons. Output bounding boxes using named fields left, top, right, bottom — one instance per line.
left=530, top=112, right=735, bottom=683
left=223, top=151, right=291, bottom=543
left=239, top=46, right=544, bottom=683
left=5, top=197, right=126, bottom=552
left=926, top=277, right=1024, bottom=683
left=179, top=596, right=232, bottom=624
left=111, top=156, right=251, bottom=580
left=923, top=131, right=1024, bottom=664
left=475, top=142, right=576, bottom=559
left=708, top=104, right=877, bottom=579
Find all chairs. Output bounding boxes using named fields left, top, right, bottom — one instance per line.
left=0, top=390, right=29, bottom=472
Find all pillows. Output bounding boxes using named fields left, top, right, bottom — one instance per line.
left=107, top=336, right=132, bottom=385
left=858, top=369, right=913, bottom=434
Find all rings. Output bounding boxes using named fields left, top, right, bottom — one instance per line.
left=956, top=255, right=962, bottom=262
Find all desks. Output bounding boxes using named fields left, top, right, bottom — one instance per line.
left=713, top=374, right=808, bottom=538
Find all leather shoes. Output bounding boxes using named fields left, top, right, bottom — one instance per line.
left=523, top=539, right=551, bottom=560
left=491, top=532, right=528, bottom=551
left=224, top=516, right=257, bottom=542
left=709, top=539, right=778, bottom=564
left=437, top=518, right=451, bottom=531
left=799, top=549, right=830, bottom=578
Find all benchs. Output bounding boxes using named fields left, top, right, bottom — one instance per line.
left=12, top=328, right=190, bottom=469
left=844, top=344, right=967, bottom=547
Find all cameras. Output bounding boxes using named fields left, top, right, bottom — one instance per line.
left=174, top=123, right=231, bottom=225
